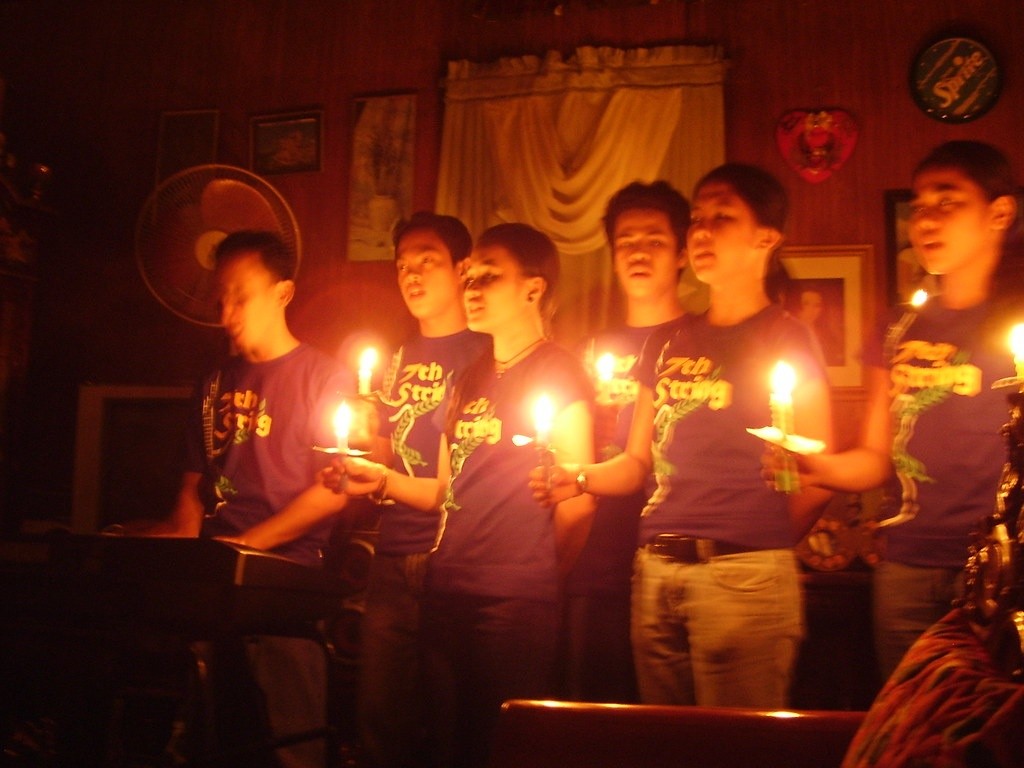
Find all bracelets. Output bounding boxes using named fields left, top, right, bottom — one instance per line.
left=368, top=463, right=395, bottom=505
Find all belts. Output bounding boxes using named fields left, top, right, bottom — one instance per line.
left=647, top=532, right=747, bottom=562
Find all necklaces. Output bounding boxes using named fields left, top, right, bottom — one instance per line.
left=493, top=335, right=548, bottom=379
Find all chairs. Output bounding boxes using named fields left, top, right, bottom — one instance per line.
left=491, top=698, right=869, bottom=768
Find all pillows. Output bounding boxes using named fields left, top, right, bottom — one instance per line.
left=842, top=607, right=1024, bottom=768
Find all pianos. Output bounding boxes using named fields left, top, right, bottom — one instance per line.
left=0, top=526, right=368, bottom=767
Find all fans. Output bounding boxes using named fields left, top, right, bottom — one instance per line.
left=134, top=165, right=303, bottom=327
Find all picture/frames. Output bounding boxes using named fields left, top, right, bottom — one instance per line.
left=779, top=244, right=878, bottom=402
left=151, top=108, right=219, bottom=227
left=885, top=189, right=945, bottom=308
left=907, top=30, right=1003, bottom=125
left=249, top=108, right=325, bottom=178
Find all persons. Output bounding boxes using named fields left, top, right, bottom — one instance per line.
left=347, top=159, right=834, bottom=768
left=140, top=231, right=367, bottom=768
left=761, top=141, right=1024, bottom=687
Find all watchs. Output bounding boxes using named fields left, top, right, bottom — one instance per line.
left=573, top=464, right=588, bottom=497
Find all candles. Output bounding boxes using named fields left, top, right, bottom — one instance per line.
left=531, top=390, right=557, bottom=520
left=768, top=359, right=799, bottom=496
left=358, top=346, right=377, bottom=394
left=334, top=402, right=353, bottom=494
